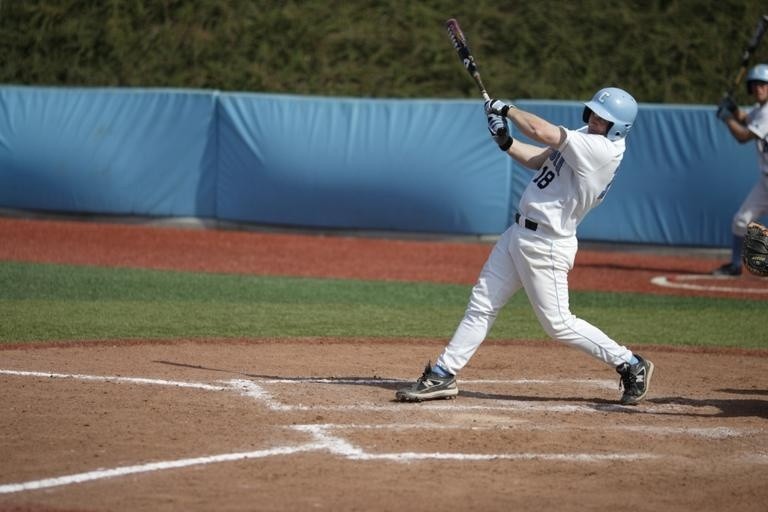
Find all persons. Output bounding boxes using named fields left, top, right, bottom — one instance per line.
left=710, top=64, right=768, bottom=277
left=395, top=86, right=656, bottom=408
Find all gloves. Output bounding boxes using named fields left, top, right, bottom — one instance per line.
left=716, top=93, right=740, bottom=123
left=487, top=114, right=513, bottom=152
left=482, top=99, right=516, bottom=118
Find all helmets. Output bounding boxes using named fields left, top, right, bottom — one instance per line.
left=581, top=86, right=638, bottom=144
left=746, top=64, right=767, bottom=95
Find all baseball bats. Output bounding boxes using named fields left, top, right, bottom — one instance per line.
left=716, top=14, right=768, bottom=118
left=447, top=19, right=507, bottom=136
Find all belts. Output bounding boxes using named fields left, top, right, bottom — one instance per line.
left=515, top=213, right=538, bottom=231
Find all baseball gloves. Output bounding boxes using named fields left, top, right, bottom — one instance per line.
left=743, top=222, right=768, bottom=276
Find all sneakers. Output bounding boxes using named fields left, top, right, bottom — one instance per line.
left=616, top=354, right=655, bottom=406
left=714, top=263, right=743, bottom=275
left=396, top=360, right=459, bottom=403
